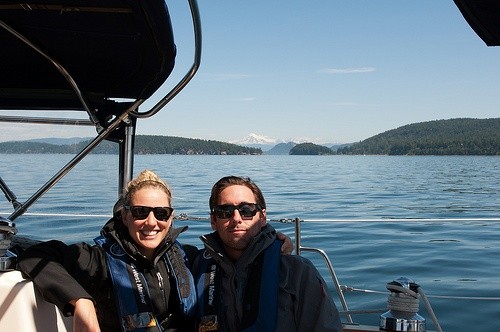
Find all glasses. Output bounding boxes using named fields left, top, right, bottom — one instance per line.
left=124, top=205, right=174, bottom=222
left=213, top=202, right=262, bottom=219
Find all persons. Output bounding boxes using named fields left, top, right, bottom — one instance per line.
left=191, top=175, right=344, bottom=332
left=17, top=168, right=295, bottom=332
